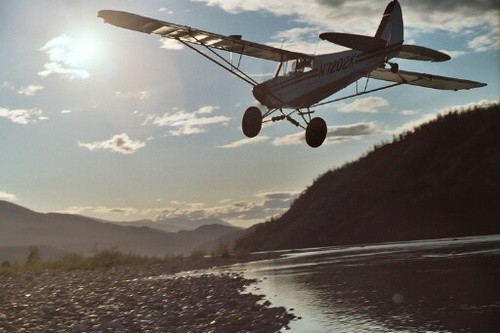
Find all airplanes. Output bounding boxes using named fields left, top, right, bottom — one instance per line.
left=97, top=0, right=487, bottom=147
